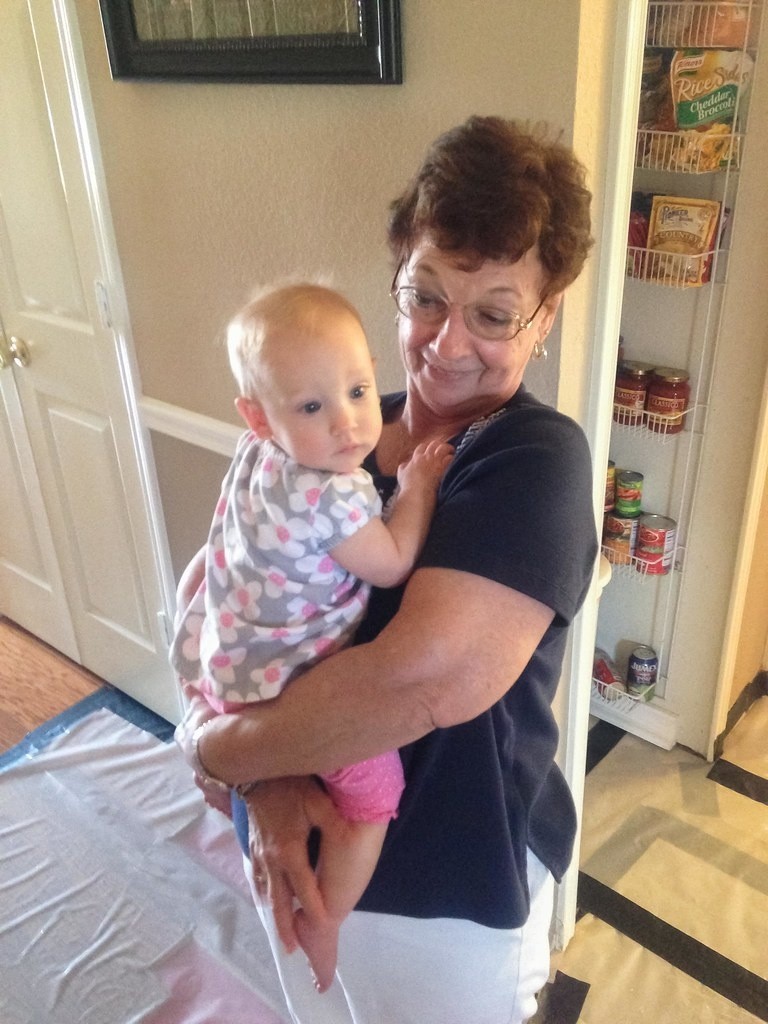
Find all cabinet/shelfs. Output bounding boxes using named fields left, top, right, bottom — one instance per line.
left=591, top=0, right=768, bottom=759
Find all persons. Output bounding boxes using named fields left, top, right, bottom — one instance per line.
left=174, top=117, right=598, bottom=1024
left=168, top=281, right=455, bottom=993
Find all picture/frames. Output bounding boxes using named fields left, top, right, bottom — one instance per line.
left=96, top=0, right=401, bottom=85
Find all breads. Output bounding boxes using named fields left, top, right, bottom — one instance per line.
left=675, top=0, right=752, bottom=49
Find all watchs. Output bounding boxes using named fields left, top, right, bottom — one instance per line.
left=191, top=719, right=228, bottom=793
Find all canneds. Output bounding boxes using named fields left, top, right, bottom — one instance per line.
left=592, top=649, right=627, bottom=700
left=602, top=459, right=677, bottom=577
left=612, top=360, right=690, bottom=434
left=625, top=646, right=659, bottom=703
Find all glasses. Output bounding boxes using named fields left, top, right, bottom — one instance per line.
left=389, top=252, right=554, bottom=342
